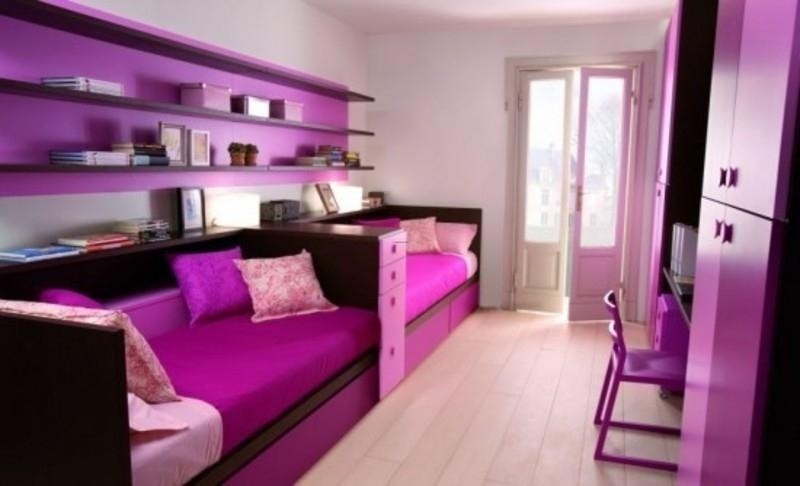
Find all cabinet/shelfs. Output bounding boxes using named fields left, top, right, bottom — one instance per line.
left=1, top=0, right=380, bottom=199
left=646, top=2, right=717, bottom=353
left=653, top=292, right=689, bottom=398
left=678, top=1, right=800, bottom=486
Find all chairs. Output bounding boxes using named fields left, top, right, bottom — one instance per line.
left=594, top=290, right=688, bottom=473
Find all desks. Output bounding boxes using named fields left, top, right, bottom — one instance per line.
left=663, top=266, right=692, bottom=325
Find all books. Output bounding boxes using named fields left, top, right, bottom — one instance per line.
left=295, top=144, right=361, bottom=169
left=669, top=220, right=697, bottom=277
left=0, top=214, right=173, bottom=266
left=48, top=140, right=170, bottom=167
left=41, top=74, right=126, bottom=97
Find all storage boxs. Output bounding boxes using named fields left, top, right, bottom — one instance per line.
left=180, top=79, right=307, bottom=123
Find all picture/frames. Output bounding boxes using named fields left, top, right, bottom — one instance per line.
left=315, top=183, right=340, bottom=213
left=154, top=121, right=214, bottom=168
left=172, top=186, right=209, bottom=236
